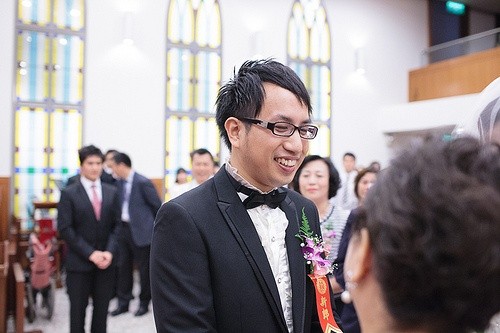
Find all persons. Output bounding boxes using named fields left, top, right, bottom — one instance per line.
left=63, top=79, right=500, bottom=306
left=108, top=152, right=164, bottom=317
left=56, top=144, right=124, bottom=333
left=289, top=155, right=362, bottom=333
left=343, top=132, right=500, bottom=332
left=148, top=56, right=345, bottom=333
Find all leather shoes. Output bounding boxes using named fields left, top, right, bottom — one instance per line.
left=111, top=306, right=128, bottom=316
left=136, top=307, right=148, bottom=316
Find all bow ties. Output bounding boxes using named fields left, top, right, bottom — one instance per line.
left=225, top=169, right=288, bottom=210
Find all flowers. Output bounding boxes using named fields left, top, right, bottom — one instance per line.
left=294, top=207, right=339, bottom=277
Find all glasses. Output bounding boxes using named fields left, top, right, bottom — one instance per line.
left=235, top=116, right=319, bottom=139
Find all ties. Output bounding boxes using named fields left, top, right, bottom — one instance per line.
left=119, top=180, right=128, bottom=212
left=92, top=183, right=101, bottom=221
